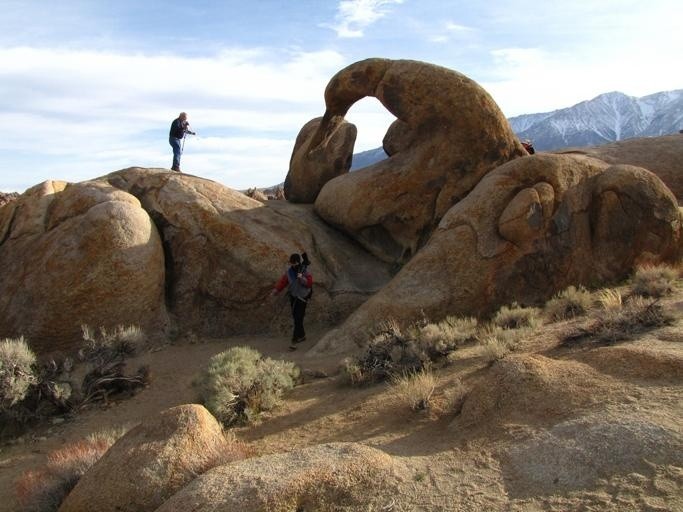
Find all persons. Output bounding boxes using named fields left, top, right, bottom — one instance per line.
left=168, top=111, right=195, bottom=172
left=521, top=139, right=534, bottom=154
left=271, top=253, right=312, bottom=349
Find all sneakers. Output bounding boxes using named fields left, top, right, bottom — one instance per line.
left=289, top=335, right=305, bottom=349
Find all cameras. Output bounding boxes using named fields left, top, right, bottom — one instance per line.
left=301, top=252, right=311, bottom=268
left=186, top=130, right=195, bottom=136
